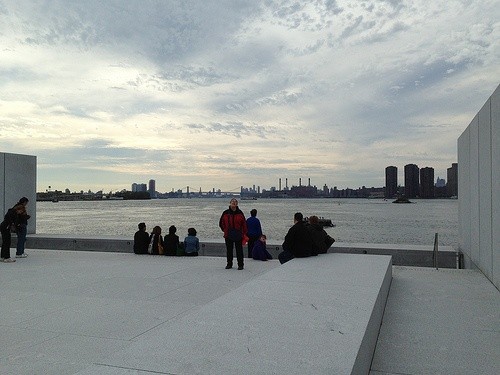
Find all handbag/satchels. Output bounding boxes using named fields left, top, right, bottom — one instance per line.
left=227, top=227, right=242, bottom=242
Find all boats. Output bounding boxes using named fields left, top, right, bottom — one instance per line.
left=392, top=195, right=413, bottom=205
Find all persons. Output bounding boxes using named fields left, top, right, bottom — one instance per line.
left=0, top=205, right=25, bottom=262
left=305, top=215, right=335, bottom=254
left=245, top=209, right=262, bottom=258
left=183, top=228, right=200, bottom=257
left=148, top=226, right=164, bottom=255
left=252, top=234, right=274, bottom=261
left=164, top=225, right=181, bottom=257
left=133, top=223, right=150, bottom=255
left=219, top=198, right=247, bottom=270
left=277, top=212, right=318, bottom=264
left=12, top=197, right=31, bottom=258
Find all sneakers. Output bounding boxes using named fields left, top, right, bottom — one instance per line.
left=0, top=257, right=5, bottom=262
left=4, top=257, right=16, bottom=263
left=16, top=253, right=28, bottom=258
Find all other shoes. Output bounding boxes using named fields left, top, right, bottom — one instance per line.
left=238, top=264, right=243, bottom=270
left=225, top=264, right=232, bottom=269
